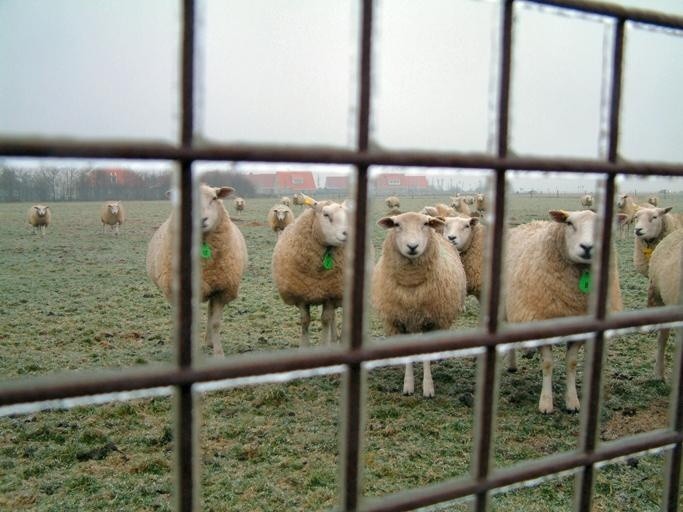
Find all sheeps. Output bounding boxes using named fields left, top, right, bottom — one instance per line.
left=269, top=191, right=376, bottom=349
left=504, top=208, right=628, bottom=415
left=233, top=193, right=246, bottom=212
left=148, top=181, right=250, bottom=361
left=642, top=225, right=683, bottom=383
left=372, top=193, right=486, bottom=399
left=580, top=194, right=594, bottom=209
left=617, top=194, right=683, bottom=281
left=26, top=205, right=54, bottom=236
left=100, top=199, right=124, bottom=234
